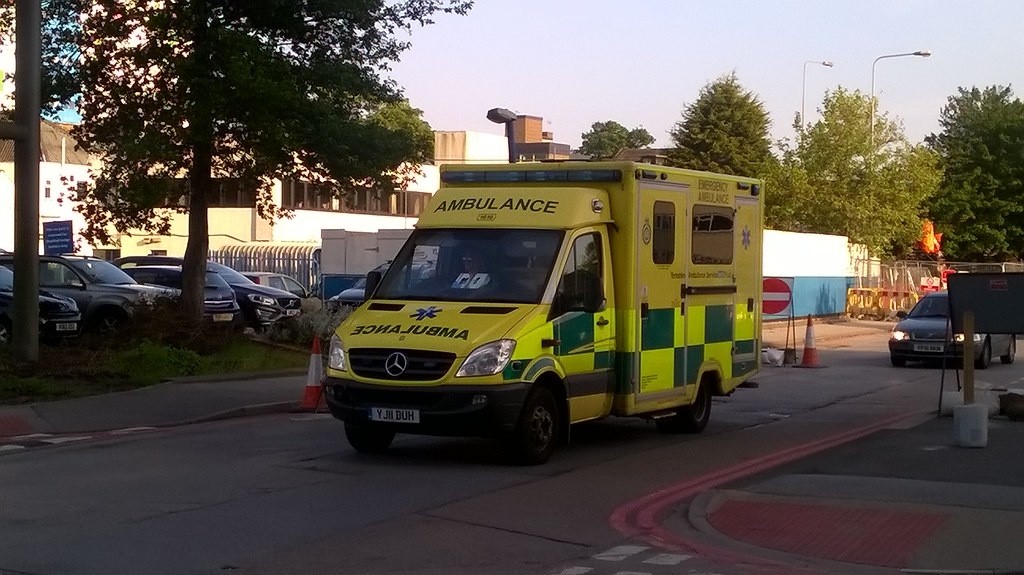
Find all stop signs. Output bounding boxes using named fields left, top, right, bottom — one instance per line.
left=761, top=277, right=792, bottom=315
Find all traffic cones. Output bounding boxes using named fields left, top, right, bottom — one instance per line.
left=791, top=314, right=829, bottom=369
left=295, top=334, right=334, bottom=415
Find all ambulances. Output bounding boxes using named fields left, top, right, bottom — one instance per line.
left=322, top=156, right=764, bottom=464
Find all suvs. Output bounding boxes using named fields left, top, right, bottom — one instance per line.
left=55, top=254, right=183, bottom=348
left=0, top=252, right=155, bottom=349
left=325, top=260, right=437, bottom=312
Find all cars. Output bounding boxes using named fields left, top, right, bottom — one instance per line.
left=0, top=264, right=82, bottom=350
left=888, top=290, right=1017, bottom=369
left=241, top=270, right=323, bottom=315
left=206, top=260, right=303, bottom=334
left=110, top=256, right=239, bottom=352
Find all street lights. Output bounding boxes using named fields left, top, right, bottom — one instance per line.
left=871, top=51, right=934, bottom=202
left=798, top=57, right=834, bottom=145
